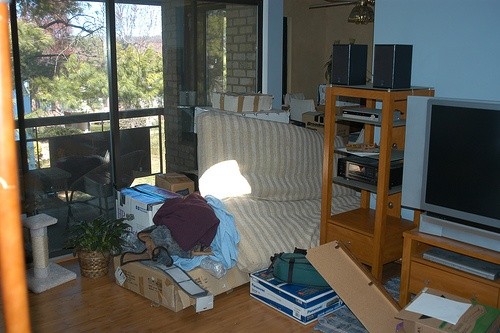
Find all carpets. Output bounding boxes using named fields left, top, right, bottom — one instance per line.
left=315, top=276, right=400, bottom=333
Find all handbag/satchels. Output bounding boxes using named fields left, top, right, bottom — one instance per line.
left=270, top=251, right=329, bottom=287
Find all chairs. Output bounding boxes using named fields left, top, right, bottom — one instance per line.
left=16, top=141, right=145, bottom=234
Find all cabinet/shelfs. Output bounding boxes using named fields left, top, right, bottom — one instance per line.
left=400, top=228, right=500, bottom=311
left=321, top=84, right=435, bottom=283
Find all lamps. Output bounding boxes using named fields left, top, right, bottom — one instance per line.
left=347, top=0, right=374, bottom=25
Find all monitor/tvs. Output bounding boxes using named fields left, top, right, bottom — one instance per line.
left=401, top=96, right=500, bottom=252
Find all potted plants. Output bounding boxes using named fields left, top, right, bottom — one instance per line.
left=61, top=215, right=136, bottom=280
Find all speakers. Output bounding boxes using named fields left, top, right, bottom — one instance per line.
left=374, top=43, right=413, bottom=88
left=331, top=44, right=368, bottom=87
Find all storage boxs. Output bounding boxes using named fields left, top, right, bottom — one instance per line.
left=193, top=106, right=289, bottom=133
left=307, top=240, right=500, bottom=333
left=113, top=252, right=251, bottom=312
left=210, top=92, right=274, bottom=112
left=115, top=184, right=182, bottom=234
left=248, top=267, right=345, bottom=324
left=155, top=173, right=194, bottom=198
left=318, top=84, right=360, bottom=107
